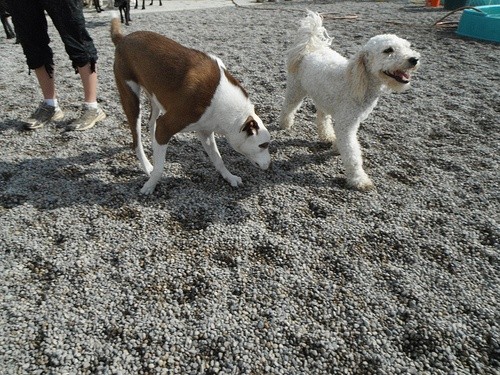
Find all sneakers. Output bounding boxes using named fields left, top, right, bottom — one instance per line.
left=65, top=102, right=106, bottom=131
left=22, top=100, right=64, bottom=129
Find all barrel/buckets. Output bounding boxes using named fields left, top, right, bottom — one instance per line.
left=425, top=0, right=440, bottom=7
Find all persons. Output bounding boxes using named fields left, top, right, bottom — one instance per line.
left=0, top=0, right=107, bottom=132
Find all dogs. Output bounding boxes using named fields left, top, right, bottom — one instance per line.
left=108, top=11, right=271, bottom=196
left=279, top=7, right=421, bottom=191
